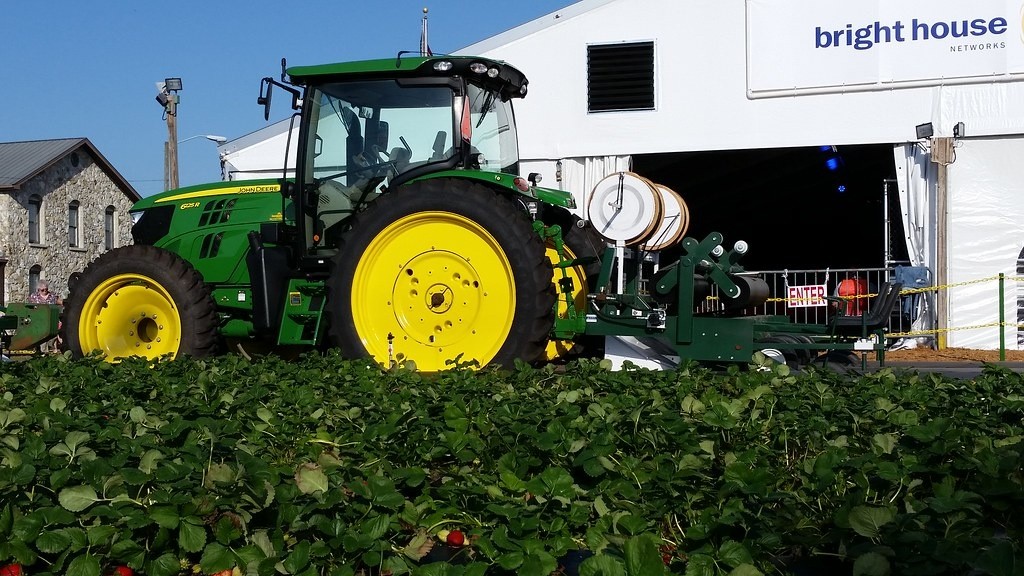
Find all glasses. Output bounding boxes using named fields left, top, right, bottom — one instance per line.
left=40, top=288, right=48, bottom=292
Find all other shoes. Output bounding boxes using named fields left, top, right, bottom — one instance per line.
left=52, top=346, right=58, bottom=353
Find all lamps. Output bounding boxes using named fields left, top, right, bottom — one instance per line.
left=952, top=121, right=964, bottom=140
left=915, top=122, right=933, bottom=140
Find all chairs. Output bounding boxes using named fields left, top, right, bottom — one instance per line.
left=400, top=131, right=447, bottom=173
left=835, top=282, right=902, bottom=326
left=838, top=282, right=891, bottom=320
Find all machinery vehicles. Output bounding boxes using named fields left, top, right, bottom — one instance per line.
left=1, top=10, right=902, bottom=386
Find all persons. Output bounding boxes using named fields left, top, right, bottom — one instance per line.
left=832, top=264, right=877, bottom=318
left=28, top=280, right=63, bottom=353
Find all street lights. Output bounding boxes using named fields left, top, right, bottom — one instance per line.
left=164, top=133, right=228, bottom=191
